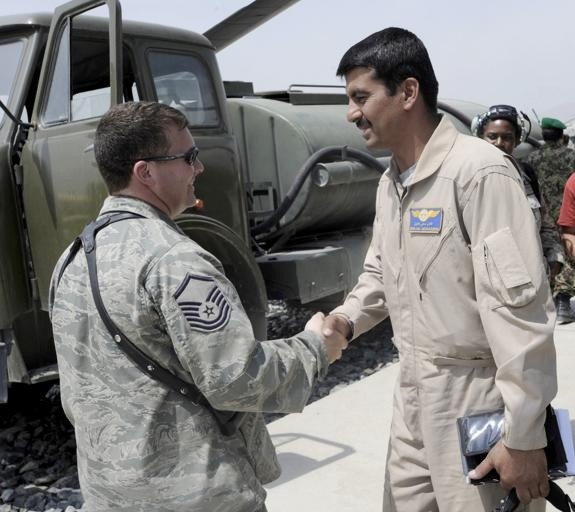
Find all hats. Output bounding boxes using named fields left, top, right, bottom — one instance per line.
left=540, top=118, right=566, bottom=130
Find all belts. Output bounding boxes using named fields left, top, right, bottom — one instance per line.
left=492, top=481, right=574, bottom=511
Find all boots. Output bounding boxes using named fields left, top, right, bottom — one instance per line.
left=557, top=293, right=575, bottom=322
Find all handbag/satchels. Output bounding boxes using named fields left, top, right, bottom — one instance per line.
left=456, top=403, right=568, bottom=486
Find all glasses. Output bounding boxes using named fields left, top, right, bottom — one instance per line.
left=133, top=147, right=199, bottom=166
